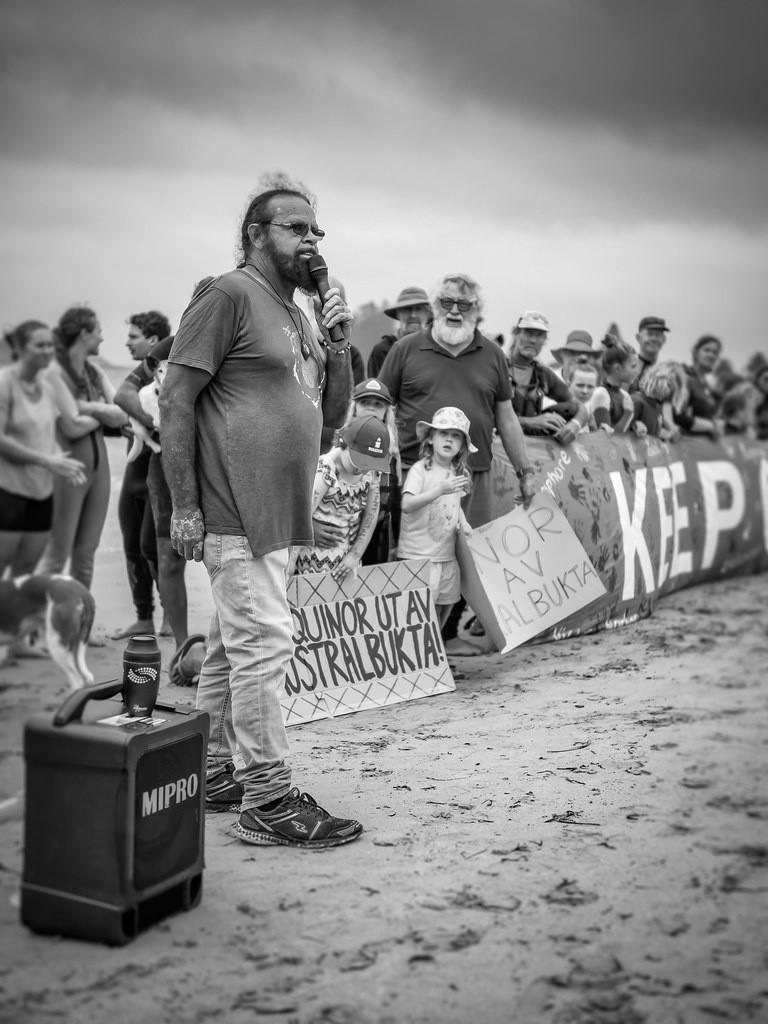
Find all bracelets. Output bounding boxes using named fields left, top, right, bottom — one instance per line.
left=323, top=339, right=352, bottom=354
left=572, top=419, right=581, bottom=429
left=517, top=467, right=534, bottom=478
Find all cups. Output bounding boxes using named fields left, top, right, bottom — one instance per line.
left=123, top=634, right=162, bottom=717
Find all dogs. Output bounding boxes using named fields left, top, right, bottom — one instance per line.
left=0, top=572, right=94, bottom=709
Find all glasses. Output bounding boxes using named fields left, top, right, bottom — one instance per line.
left=439, top=297, right=474, bottom=312
left=264, top=221, right=325, bottom=241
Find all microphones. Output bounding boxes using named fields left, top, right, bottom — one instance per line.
left=307, top=254, right=345, bottom=341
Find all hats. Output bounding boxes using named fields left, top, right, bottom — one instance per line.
left=341, top=414, right=391, bottom=472
left=638, top=316, right=670, bottom=332
left=384, top=286, right=431, bottom=320
left=517, top=311, right=550, bottom=333
left=353, top=376, right=394, bottom=405
left=551, top=330, right=603, bottom=364
left=416, top=407, right=479, bottom=452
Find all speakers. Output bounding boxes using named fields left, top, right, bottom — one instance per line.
left=21, top=678, right=211, bottom=948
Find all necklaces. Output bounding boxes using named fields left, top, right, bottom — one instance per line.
left=246, top=263, right=310, bottom=360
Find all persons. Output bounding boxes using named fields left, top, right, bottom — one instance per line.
left=155, top=189, right=365, bottom=848
left=495, top=311, right=768, bottom=446
left=280, top=273, right=535, bottom=658
left=0, top=307, right=189, bottom=658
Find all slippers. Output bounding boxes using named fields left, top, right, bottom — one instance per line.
left=168, top=634, right=207, bottom=687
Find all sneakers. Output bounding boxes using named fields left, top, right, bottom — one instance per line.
left=233, top=787, right=363, bottom=848
left=206, top=761, right=245, bottom=812
